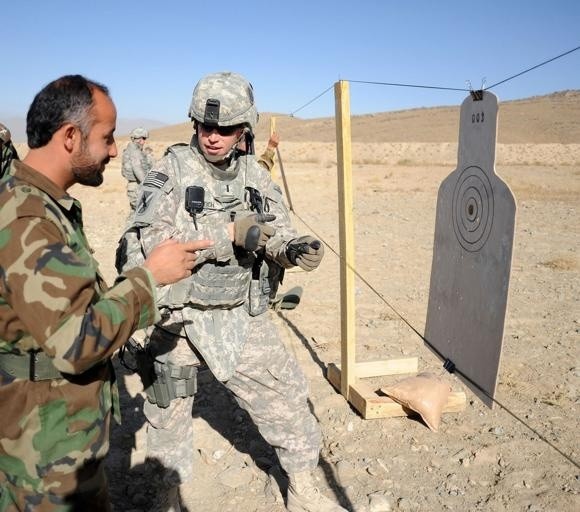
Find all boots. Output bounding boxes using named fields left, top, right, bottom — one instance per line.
left=286, top=473, right=350, bottom=512
left=149, top=486, right=181, bottom=512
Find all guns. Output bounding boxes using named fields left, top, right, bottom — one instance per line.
left=126, top=336, right=147, bottom=368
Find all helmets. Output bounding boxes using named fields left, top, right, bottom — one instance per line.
left=131, top=128, right=149, bottom=139
left=188, top=71, right=258, bottom=129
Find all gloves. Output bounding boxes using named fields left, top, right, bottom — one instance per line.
left=286, top=235, right=324, bottom=272
left=233, top=213, right=277, bottom=252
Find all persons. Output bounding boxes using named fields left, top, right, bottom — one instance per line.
left=0, top=72, right=214, bottom=512
left=120, top=126, right=154, bottom=215
left=114, top=72, right=347, bottom=512
left=256, top=129, right=281, bottom=173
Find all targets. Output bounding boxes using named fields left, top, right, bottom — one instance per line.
left=422, top=90, right=516, bottom=411
